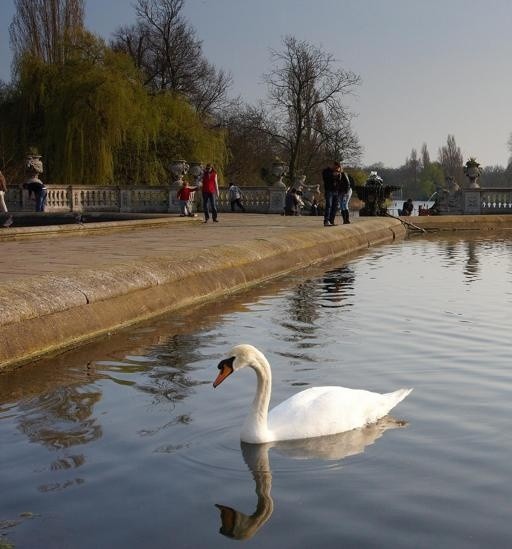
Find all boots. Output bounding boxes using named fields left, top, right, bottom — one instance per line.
left=342, top=212, right=350, bottom=224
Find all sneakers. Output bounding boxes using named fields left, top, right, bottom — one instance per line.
left=324, top=220, right=333, bottom=226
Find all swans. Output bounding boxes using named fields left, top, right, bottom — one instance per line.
left=213, top=344, right=415, bottom=444
left=213, top=415, right=411, bottom=543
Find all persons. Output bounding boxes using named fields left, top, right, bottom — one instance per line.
left=322, top=161, right=341, bottom=226
left=336, top=165, right=353, bottom=224
left=1, top=172, right=15, bottom=227
left=176, top=180, right=198, bottom=217
left=400, top=198, right=414, bottom=216
left=296, top=191, right=305, bottom=216
left=227, top=182, right=246, bottom=213
left=22, top=182, right=49, bottom=213
left=285, top=188, right=300, bottom=216
left=196, top=162, right=220, bottom=224
